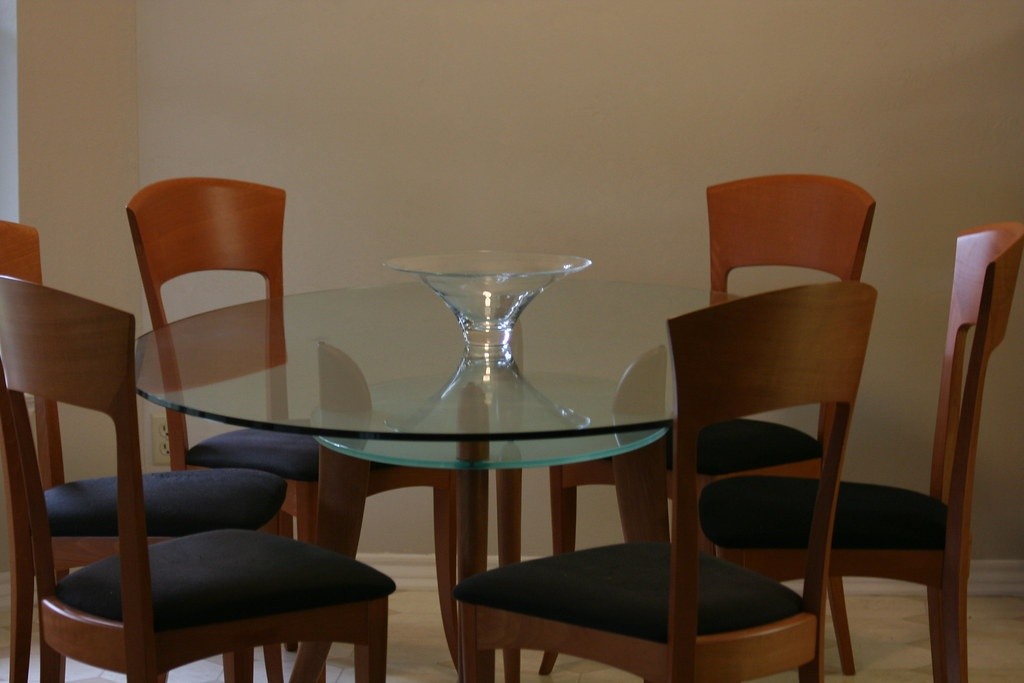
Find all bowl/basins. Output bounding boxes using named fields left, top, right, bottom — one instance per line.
left=378, top=249, right=593, bottom=343
left=382, top=338, right=591, bottom=434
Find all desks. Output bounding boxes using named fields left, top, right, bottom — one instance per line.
left=134, top=279, right=747, bottom=683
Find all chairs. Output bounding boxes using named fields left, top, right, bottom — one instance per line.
left=123, top=175, right=461, bottom=683
left=0, top=272, right=397, bottom=683
left=695, top=220, right=1024, bottom=683
left=455, top=276, right=879, bottom=683
left=0, top=222, right=289, bottom=683
left=532, top=173, right=877, bottom=675
